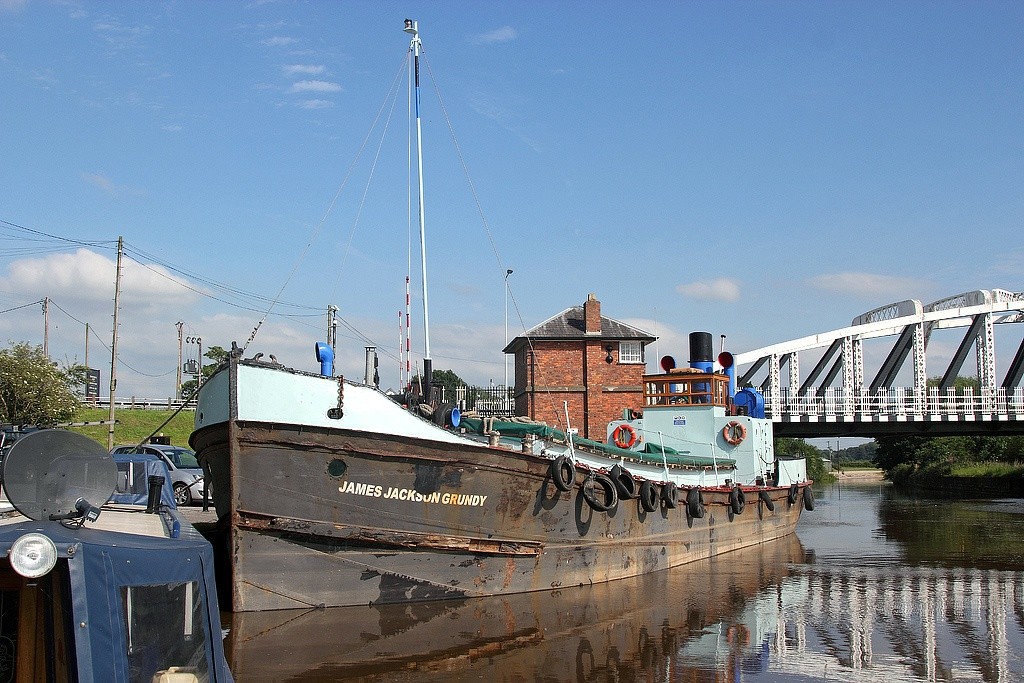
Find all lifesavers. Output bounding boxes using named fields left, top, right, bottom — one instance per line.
left=582, top=473, right=617, bottom=512
left=606, top=647, right=621, bottom=683
left=689, top=609, right=704, bottom=637
left=642, top=637, right=658, bottom=670
left=577, top=639, right=596, bottom=683
left=731, top=487, right=745, bottom=515
left=664, top=629, right=678, bottom=656
left=789, top=484, right=799, bottom=504
left=610, top=464, right=636, bottom=500
left=551, top=455, right=575, bottom=490
left=803, top=487, right=814, bottom=511
left=727, top=625, right=750, bottom=650
left=687, top=489, right=704, bottom=518
left=640, top=479, right=659, bottom=512
left=759, top=491, right=774, bottom=511
left=723, top=422, right=746, bottom=445
left=614, top=425, right=636, bottom=448
left=663, top=482, right=679, bottom=509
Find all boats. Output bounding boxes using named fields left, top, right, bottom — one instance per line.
left=0, top=426, right=236, bottom=683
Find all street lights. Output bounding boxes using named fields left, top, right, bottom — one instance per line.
left=505, top=269, right=514, bottom=416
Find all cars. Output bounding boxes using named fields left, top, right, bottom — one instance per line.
left=108, top=443, right=214, bottom=507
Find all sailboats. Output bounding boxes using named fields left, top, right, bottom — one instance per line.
left=186, top=18, right=814, bottom=613
